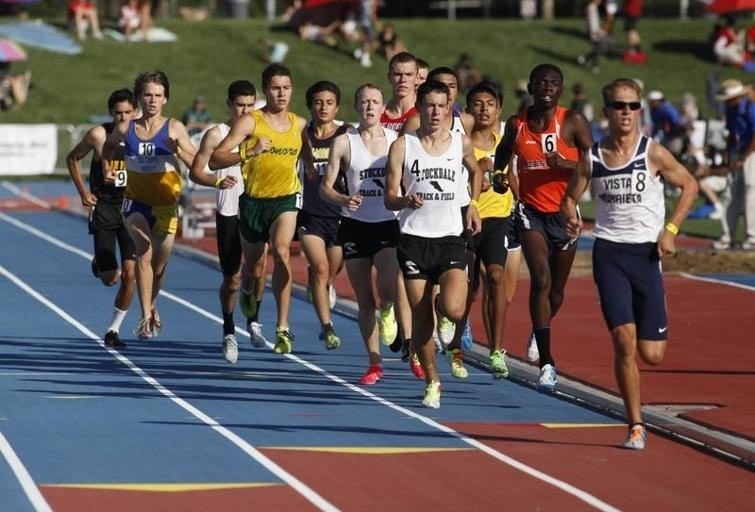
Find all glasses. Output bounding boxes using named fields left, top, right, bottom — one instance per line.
left=606, top=101, right=642, bottom=111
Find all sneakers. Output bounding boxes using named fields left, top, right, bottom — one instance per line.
left=389, top=323, right=403, bottom=353
left=444, top=347, right=469, bottom=379
left=149, top=310, right=162, bottom=338
left=526, top=333, right=539, bottom=362
left=422, top=381, right=443, bottom=410
left=711, top=241, right=735, bottom=250
left=246, top=322, right=267, bottom=348
left=318, top=327, right=341, bottom=350
left=273, top=326, right=295, bottom=354
left=379, top=303, right=398, bottom=346
left=222, top=333, right=239, bottom=365
left=459, top=315, right=473, bottom=354
left=741, top=240, right=755, bottom=249
left=707, top=211, right=725, bottom=221
left=434, top=293, right=456, bottom=345
left=136, top=318, right=153, bottom=341
left=91, top=256, right=99, bottom=278
left=408, top=339, right=427, bottom=379
left=536, top=364, right=558, bottom=393
left=623, top=422, right=648, bottom=450
left=306, top=286, right=313, bottom=302
left=431, top=332, right=443, bottom=355
left=326, top=284, right=337, bottom=312
left=400, top=336, right=412, bottom=363
left=103, top=330, right=127, bottom=351
left=358, top=364, right=384, bottom=385
left=488, top=349, right=509, bottom=380
left=239, top=284, right=258, bottom=319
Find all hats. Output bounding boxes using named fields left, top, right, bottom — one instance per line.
left=647, top=91, right=665, bottom=102
left=714, top=78, right=751, bottom=102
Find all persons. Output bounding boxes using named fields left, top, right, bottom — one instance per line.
left=1, top=2, right=754, bottom=448
